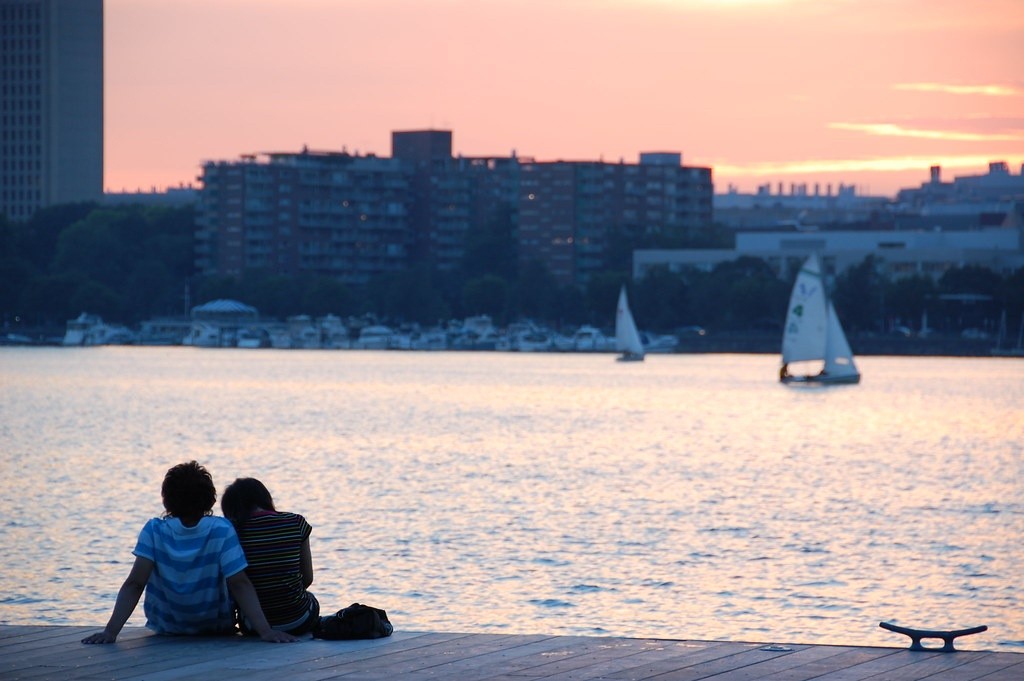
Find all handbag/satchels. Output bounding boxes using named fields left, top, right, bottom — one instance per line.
left=312, top=603, right=393, bottom=640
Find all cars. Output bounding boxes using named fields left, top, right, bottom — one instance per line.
left=1, top=315, right=715, bottom=349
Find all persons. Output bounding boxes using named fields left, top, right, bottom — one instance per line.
left=221, top=478, right=320, bottom=638
left=81, top=460, right=301, bottom=644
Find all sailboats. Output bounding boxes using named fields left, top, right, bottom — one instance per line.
left=779, top=251, right=861, bottom=385
left=616, top=282, right=648, bottom=363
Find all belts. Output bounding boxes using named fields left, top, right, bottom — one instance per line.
left=241, top=601, right=313, bottom=631
